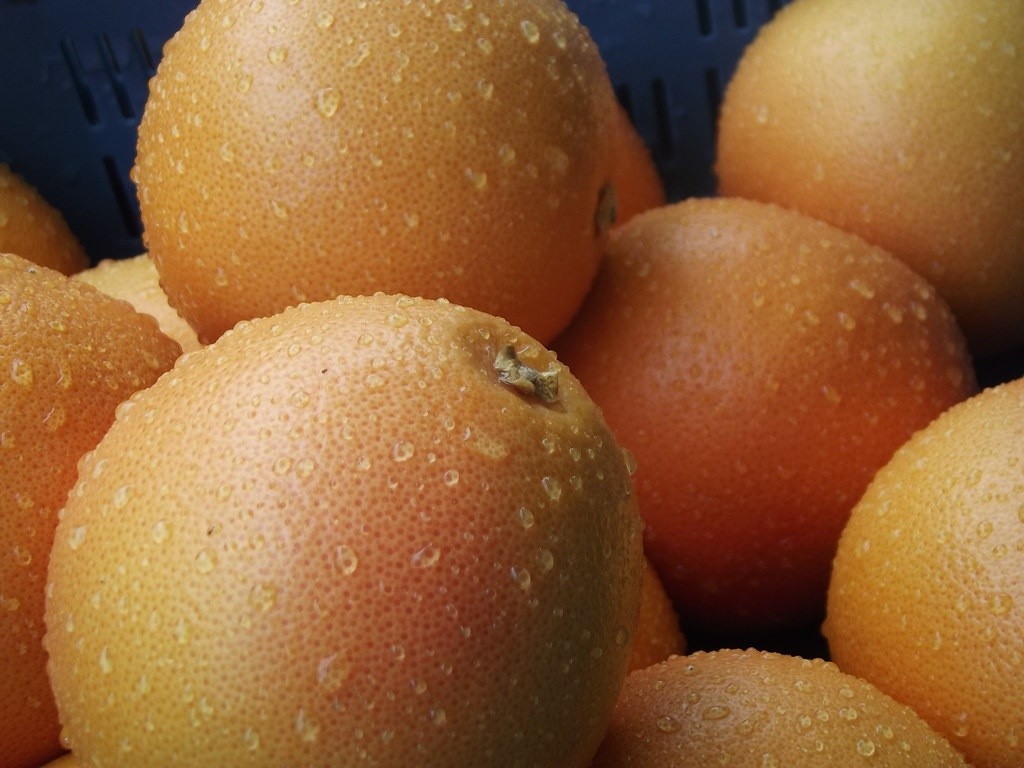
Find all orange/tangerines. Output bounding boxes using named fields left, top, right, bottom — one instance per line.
left=0, top=0, right=1024, bottom=768
